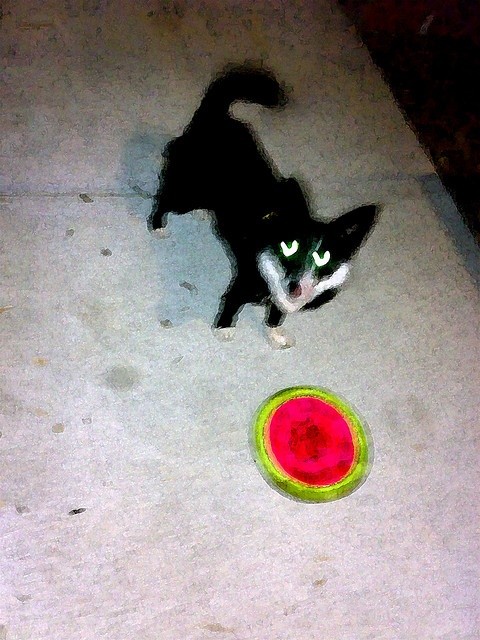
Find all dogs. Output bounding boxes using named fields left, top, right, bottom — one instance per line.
left=147, top=62, right=381, bottom=347
